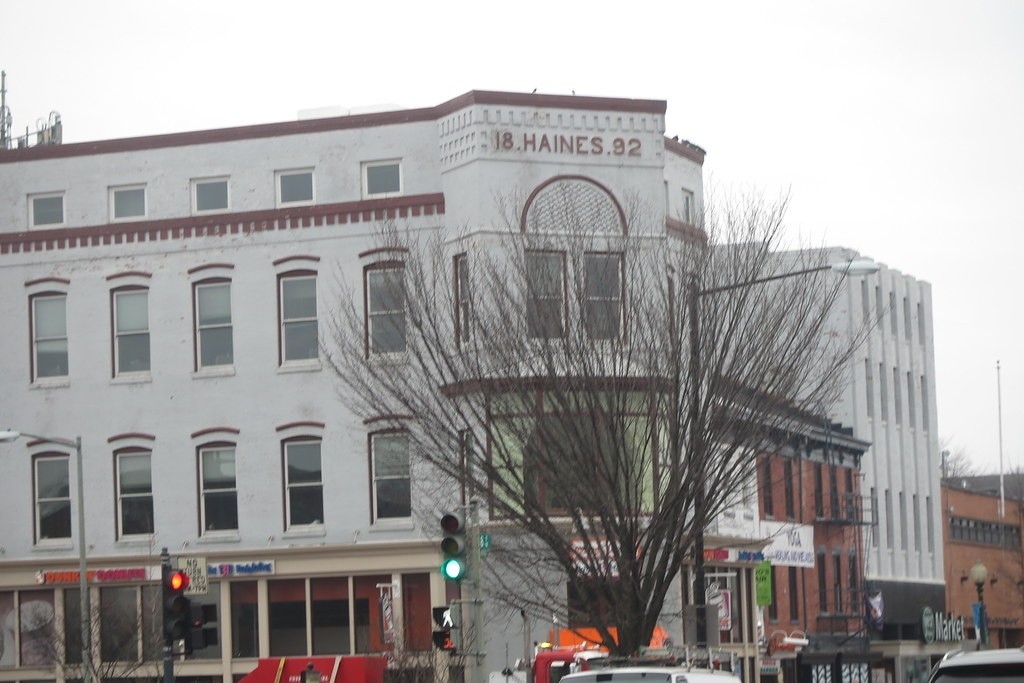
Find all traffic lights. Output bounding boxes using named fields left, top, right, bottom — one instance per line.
left=439, top=511, right=468, bottom=581
left=431, top=605, right=455, bottom=650
left=162, top=564, right=190, bottom=639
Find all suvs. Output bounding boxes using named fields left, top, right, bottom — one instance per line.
left=931, top=648, right=1024, bottom=683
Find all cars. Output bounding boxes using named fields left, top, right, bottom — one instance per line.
left=556, top=668, right=740, bottom=682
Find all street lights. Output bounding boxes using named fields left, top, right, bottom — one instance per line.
left=969, top=559, right=991, bottom=648
